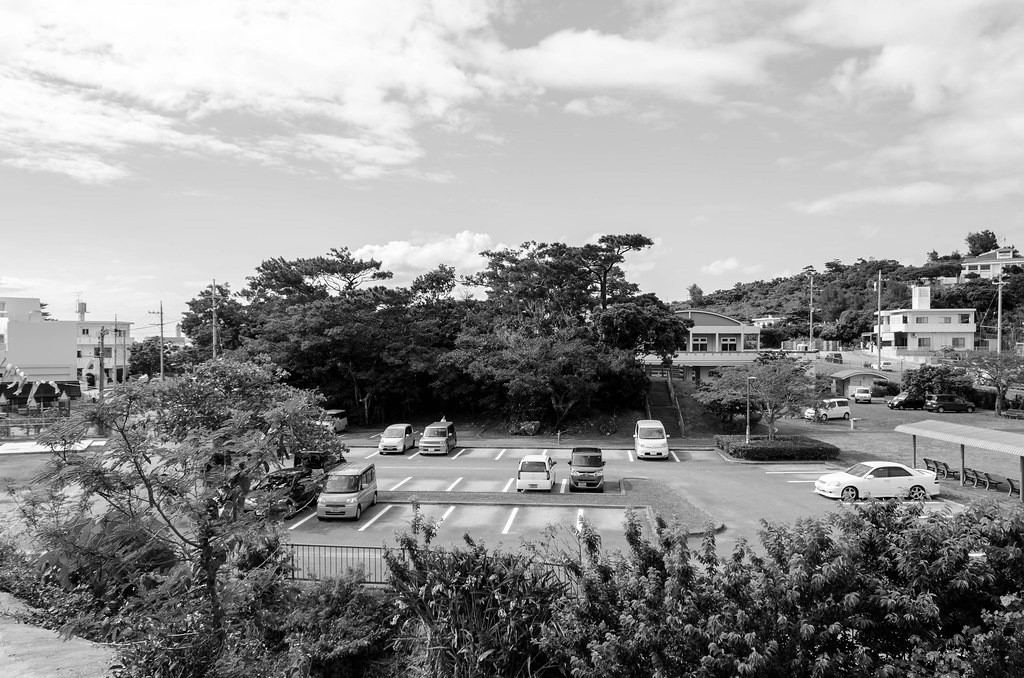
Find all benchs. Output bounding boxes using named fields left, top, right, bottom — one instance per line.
left=923, top=457, right=960, bottom=480
left=1006, top=477, right=1021, bottom=496
left=963, top=467, right=1003, bottom=491
left=1001, top=408, right=1024, bottom=420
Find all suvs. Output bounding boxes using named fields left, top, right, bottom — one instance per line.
left=854, top=388, right=872, bottom=404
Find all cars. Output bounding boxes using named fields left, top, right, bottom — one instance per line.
left=871, top=361, right=892, bottom=371
left=379, top=424, right=415, bottom=455
left=516, top=455, right=557, bottom=493
left=893, top=392, right=914, bottom=401
left=887, top=394, right=925, bottom=410
left=814, top=461, right=940, bottom=503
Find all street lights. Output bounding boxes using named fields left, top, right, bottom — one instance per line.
left=745, top=376, right=757, bottom=444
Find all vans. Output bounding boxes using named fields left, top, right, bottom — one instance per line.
left=293, top=450, right=347, bottom=472
left=419, top=422, right=457, bottom=455
left=244, top=468, right=321, bottom=520
left=824, top=353, right=842, bottom=363
left=316, top=409, right=347, bottom=435
left=317, top=461, right=378, bottom=521
left=805, top=398, right=850, bottom=421
left=925, top=394, right=975, bottom=413
left=632, top=419, right=670, bottom=460
left=568, top=447, right=607, bottom=492
